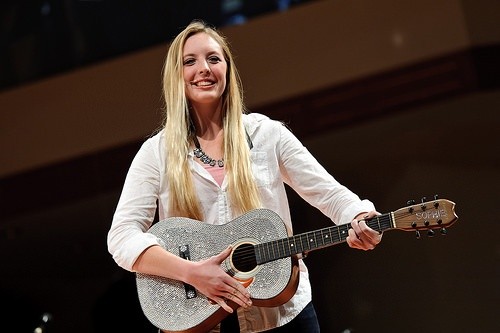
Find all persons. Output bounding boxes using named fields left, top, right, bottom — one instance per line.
left=107, top=22, right=386, bottom=333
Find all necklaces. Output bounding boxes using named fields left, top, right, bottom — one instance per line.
left=188, top=124, right=224, bottom=168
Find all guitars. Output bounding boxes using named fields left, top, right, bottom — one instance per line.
left=136, top=195, right=458, bottom=333
